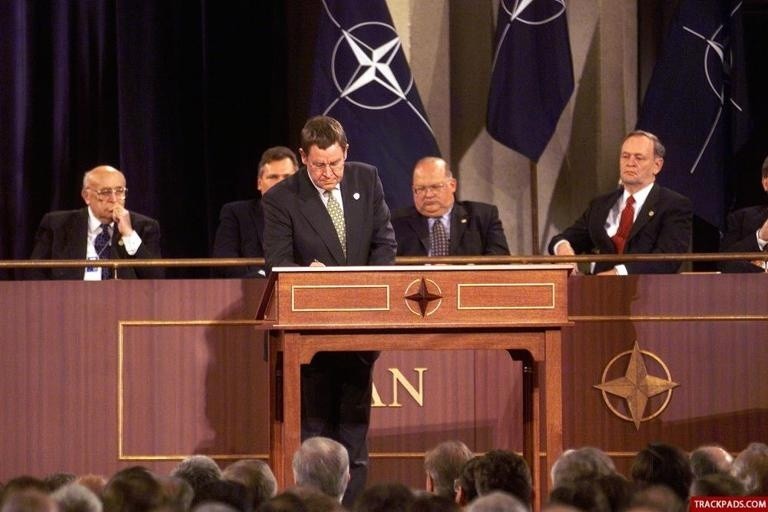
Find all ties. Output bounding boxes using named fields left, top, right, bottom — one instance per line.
left=430, top=219, right=449, bottom=257
left=324, top=191, right=347, bottom=256
left=95, top=224, right=114, bottom=279
left=611, top=196, right=636, bottom=254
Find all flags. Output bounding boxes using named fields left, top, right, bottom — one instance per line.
left=297, top=0, right=460, bottom=216
left=633, top=0, right=754, bottom=238
left=484, top=0, right=575, bottom=166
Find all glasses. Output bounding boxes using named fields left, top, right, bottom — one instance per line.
left=91, top=187, right=128, bottom=201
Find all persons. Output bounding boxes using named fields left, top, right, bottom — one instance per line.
left=213, top=144, right=299, bottom=278
left=391, top=155, right=511, bottom=264
left=25, top=164, right=166, bottom=280
left=261, top=116, right=398, bottom=503
left=0, top=435, right=768, bottom=512
left=718, top=156, right=768, bottom=274
left=548, top=128, right=696, bottom=275
